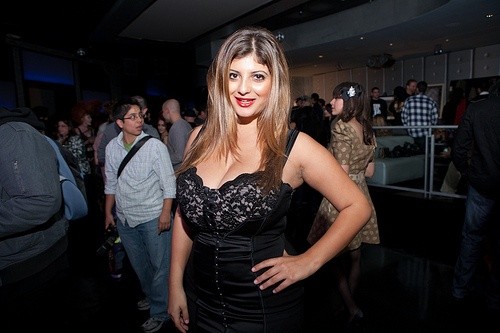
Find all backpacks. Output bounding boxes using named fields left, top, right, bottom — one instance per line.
left=43, top=133, right=88, bottom=221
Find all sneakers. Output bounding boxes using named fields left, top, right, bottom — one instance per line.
left=136, top=299, right=151, bottom=311
left=141, top=317, right=163, bottom=332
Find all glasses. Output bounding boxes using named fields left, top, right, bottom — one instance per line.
left=122, top=113, right=145, bottom=121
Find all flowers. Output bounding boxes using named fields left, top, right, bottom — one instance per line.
left=347, top=86, right=356, bottom=97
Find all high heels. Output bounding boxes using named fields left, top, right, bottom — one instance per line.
left=346, top=310, right=364, bottom=328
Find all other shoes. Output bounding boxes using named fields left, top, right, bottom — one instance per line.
left=114, top=274, right=122, bottom=278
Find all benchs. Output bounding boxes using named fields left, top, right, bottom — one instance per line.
left=366, top=135, right=439, bottom=185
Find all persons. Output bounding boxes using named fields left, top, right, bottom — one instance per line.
left=371, top=77, right=500, bottom=325
left=104, top=97, right=181, bottom=333
left=287, top=90, right=334, bottom=148
left=167, top=28, right=372, bottom=333
left=305, top=82, right=380, bottom=320
left=0, top=94, right=207, bottom=333
